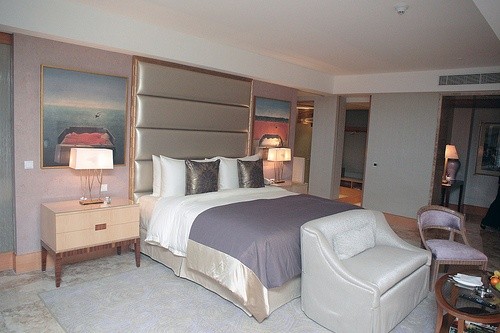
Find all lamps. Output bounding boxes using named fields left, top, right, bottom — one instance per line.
left=69, top=148, right=114, bottom=205
left=267, top=148, right=291, bottom=184
left=442, top=144, right=459, bottom=184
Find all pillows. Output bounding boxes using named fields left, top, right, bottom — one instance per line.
left=184, top=159, right=220, bottom=196
left=218, top=154, right=259, bottom=191
left=157, top=155, right=220, bottom=200
left=151, top=154, right=161, bottom=197
left=236, top=158, right=265, bottom=188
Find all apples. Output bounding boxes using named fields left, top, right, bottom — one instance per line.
left=490, top=278, right=500, bottom=291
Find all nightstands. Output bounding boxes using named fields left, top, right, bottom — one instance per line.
left=40, top=197, right=141, bottom=287
left=276, top=180, right=308, bottom=194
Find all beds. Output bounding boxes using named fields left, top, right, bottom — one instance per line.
left=129, top=55, right=365, bottom=324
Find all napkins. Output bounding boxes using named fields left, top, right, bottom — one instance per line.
left=452, top=272, right=483, bottom=287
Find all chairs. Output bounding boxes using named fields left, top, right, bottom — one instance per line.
left=416, top=205, right=488, bottom=293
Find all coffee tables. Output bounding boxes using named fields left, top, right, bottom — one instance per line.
left=435, top=269, right=500, bottom=333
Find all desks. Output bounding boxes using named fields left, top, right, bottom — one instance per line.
left=441, top=180, right=465, bottom=212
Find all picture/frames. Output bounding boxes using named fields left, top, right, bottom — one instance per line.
left=252, top=96, right=291, bottom=154
left=39, top=63, right=129, bottom=169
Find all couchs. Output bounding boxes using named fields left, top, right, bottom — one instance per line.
left=300, top=209, right=432, bottom=333
